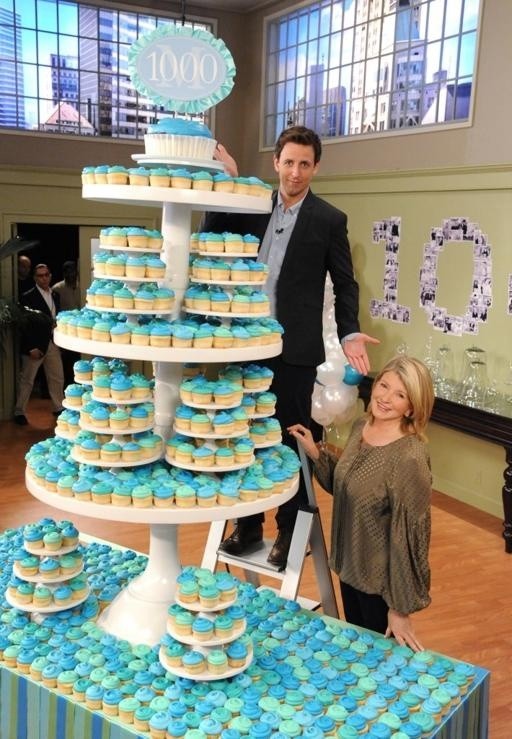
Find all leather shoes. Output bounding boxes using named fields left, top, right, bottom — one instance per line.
left=15, top=414, right=29, bottom=425
left=220, top=521, right=264, bottom=556
left=264, top=530, right=293, bottom=565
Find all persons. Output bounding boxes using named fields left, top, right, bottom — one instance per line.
left=198, top=126, right=383, bottom=565
left=285, top=355, right=442, bottom=653
left=12, top=263, right=65, bottom=425
left=369, top=216, right=492, bottom=335
left=17, top=254, right=35, bottom=294
left=51, top=260, right=83, bottom=391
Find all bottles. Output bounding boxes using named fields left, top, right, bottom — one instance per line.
left=398, top=336, right=512, bottom=405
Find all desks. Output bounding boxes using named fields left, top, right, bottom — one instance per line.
left=0, top=519, right=491, bottom=739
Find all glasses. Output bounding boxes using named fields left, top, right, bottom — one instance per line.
left=36, top=272, right=50, bottom=278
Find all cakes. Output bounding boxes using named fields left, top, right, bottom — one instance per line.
left=56, top=307, right=284, bottom=348
left=24, top=439, right=301, bottom=509
left=143, top=118, right=217, bottom=158
left=165, top=375, right=254, bottom=468
left=85, top=225, right=176, bottom=310
left=4, top=518, right=90, bottom=608
left=74, top=373, right=164, bottom=462
left=81, top=165, right=275, bottom=202
left=160, top=568, right=254, bottom=679
left=183, top=230, right=274, bottom=313
left=219, top=364, right=283, bottom=445
left=56, top=356, right=128, bottom=439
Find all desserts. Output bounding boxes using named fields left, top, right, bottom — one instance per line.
left=0, top=529, right=475, bottom=739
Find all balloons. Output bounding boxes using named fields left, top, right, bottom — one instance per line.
left=304, top=270, right=367, bottom=427
